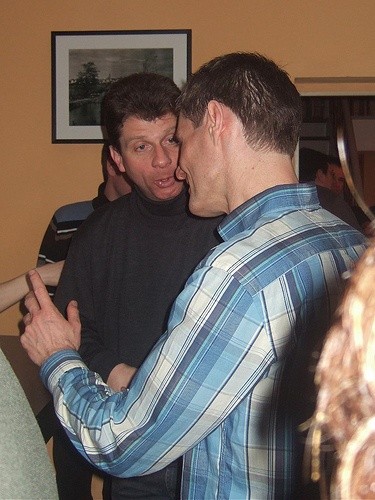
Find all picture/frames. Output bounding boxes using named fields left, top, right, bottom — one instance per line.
left=51, top=29, right=192, bottom=144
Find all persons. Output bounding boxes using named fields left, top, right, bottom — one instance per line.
left=18, top=50, right=372, bottom=499
left=0, top=258, right=66, bottom=314
left=297, top=147, right=364, bottom=234
left=301, top=219, right=375, bottom=500
left=324, top=153, right=348, bottom=194
left=36, top=143, right=142, bottom=500
left=48, top=70, right=230, bottom=500
